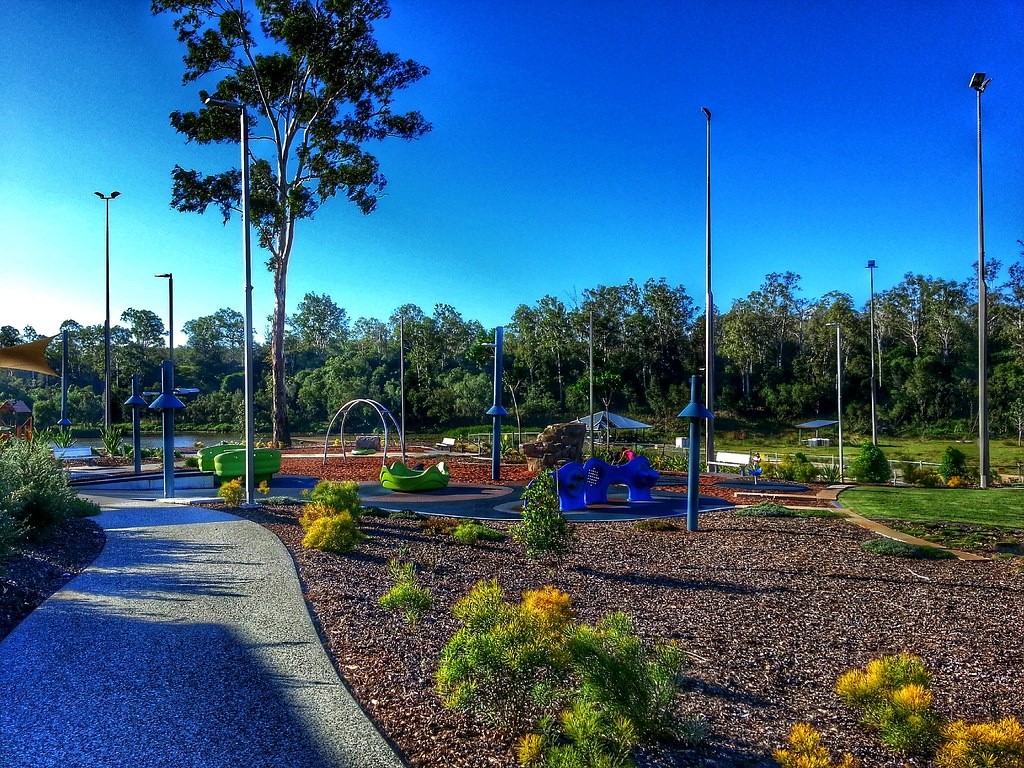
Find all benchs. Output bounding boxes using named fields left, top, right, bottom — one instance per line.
left=708, top=451, right=753, bottom=476
left=436, top=438, right=456, bottom=452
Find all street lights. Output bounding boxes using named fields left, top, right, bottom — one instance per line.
left=970, top=72, right=993, bottom=488
left=701, top=108, right=717, bottom=472
left=826, top=322, right=843, bottom=482
left=94, top=191, right=121, bottom=439
left=396, top=314, right=408, bottom=457
left=865, top=260, right=878, bottom=447
left=205, top=97, right=258, bottom=505
left=155, top=273, right=174, bottom=359
left=482, top=326, right=508, bottom=481
left=589, top=311, right=596, bottom=457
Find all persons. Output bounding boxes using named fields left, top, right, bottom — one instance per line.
left=606, top=446, right=637, bottom=467
left=751, top=452, right=761, bottom=472
